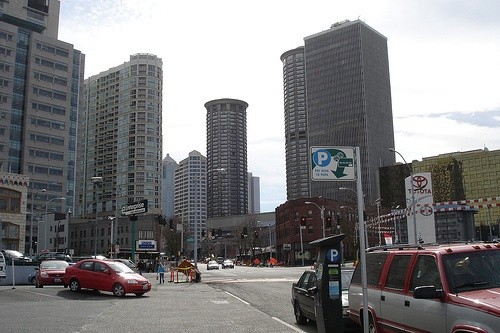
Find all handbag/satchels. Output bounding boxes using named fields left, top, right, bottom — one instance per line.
left=156, top=276, right=158, bottom=280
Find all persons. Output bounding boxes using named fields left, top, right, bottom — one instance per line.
left=159, top=263, right=165, bottom=284
left=138, top=261, right=143, bottom=275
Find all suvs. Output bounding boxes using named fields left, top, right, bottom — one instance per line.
left=347, top=240, right=500, bottom=333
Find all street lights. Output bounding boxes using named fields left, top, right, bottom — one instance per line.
left=257, top=221, right=272, bottom=258
left=46, top=197, right=66, bottom=252
left=389, top=148, right=417, bottom=245
left=340, top=187, right=369, bottom=250
left=304, top=202, right=325, bottom=238
left=193, top=168, right=225, bottom=267
left=30, top=188, right=46, bottom=258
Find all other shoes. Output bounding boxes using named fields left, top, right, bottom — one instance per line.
left=162, top=280, right=164, bottom=283
left=160, top=282, right=161, bottom=284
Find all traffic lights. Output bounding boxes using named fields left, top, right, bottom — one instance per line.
left=300, top=215, right=306, bottom=226
left=327, top=217, right=331, bottom=226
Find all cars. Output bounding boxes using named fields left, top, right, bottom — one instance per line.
left=290, top=270, right=355, bottom=324
left=2, top=250, right=33, bottom=265
left=35, top=260, right=70, bottom=288
left=92, top=255, right=140, bottom=274
left=207, top=261, right=219, bottom=270
left=64, top=260, right=151, bottom=298
left=33, top=252, right=74, bottom=263
left=0, top=252, right=7, bottom=278
left=222, top=259, right=234, bottom=269
